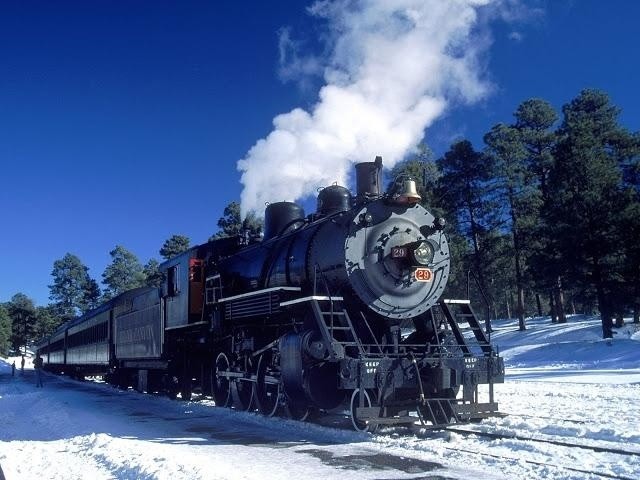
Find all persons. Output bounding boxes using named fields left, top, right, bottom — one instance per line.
left=32, top=353, right=44, bottom=388
left=20, top=355, right=25, bottom=371
left=11, top=360, right=16, bottom=377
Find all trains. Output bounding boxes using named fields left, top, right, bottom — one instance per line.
left=31, top=158, right=506, bottom=433
